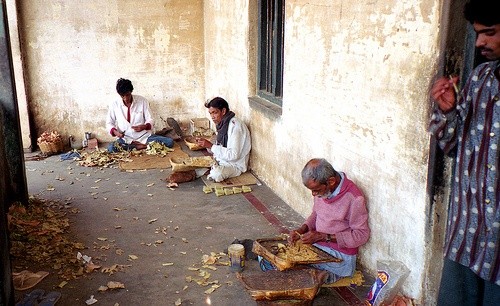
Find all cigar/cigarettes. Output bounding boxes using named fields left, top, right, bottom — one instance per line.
left=450, top=75, right=458, bottom=93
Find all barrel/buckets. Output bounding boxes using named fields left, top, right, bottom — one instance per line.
left=228, top=244, right=245, bottom=273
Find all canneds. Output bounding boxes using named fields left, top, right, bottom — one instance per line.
left=228, top=243, right=245, bottom=273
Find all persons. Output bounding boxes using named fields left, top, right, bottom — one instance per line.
left=106, top=78, right=174, bottom=153
left=194, top=97, right=251, bottom=182
left=427, top=0, right=500, bottom=306
left=258, top=159, right=370, bottom=284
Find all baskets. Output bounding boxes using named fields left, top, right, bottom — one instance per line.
left=39, top=139, right=64, bottom=156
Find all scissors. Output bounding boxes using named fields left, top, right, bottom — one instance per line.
left=270, top=245, right=286, bottom=256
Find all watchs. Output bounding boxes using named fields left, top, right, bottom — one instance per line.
left=326, top=233, right=331, bottom=244
